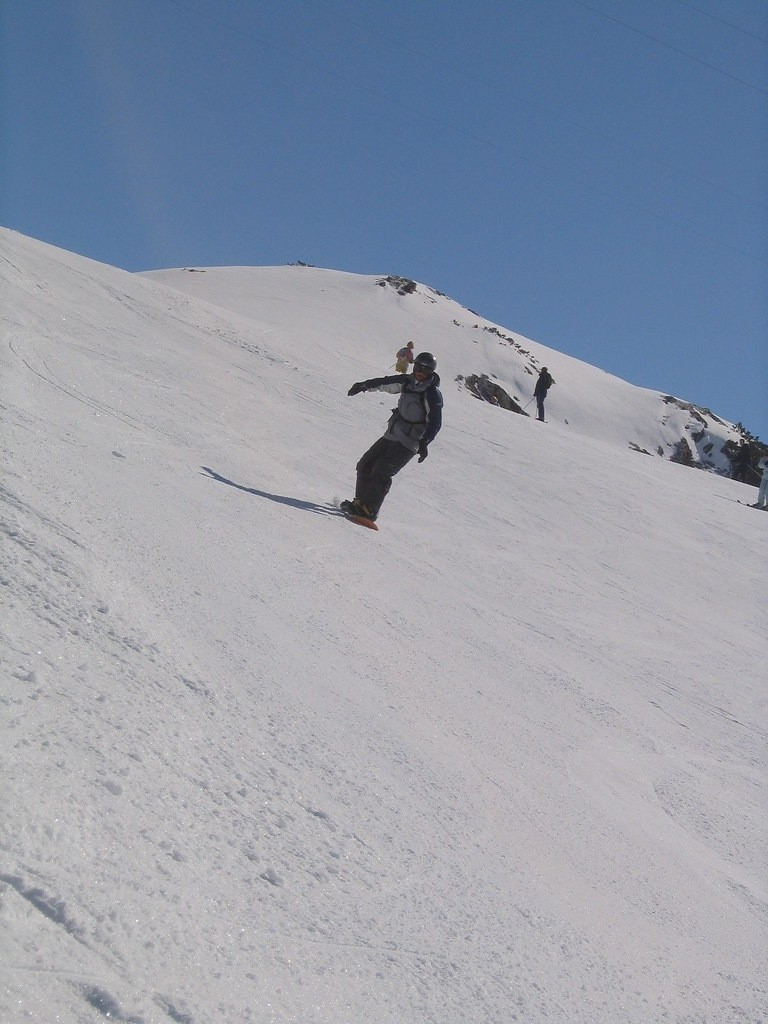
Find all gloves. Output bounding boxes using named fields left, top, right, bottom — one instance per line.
left=348, top=381, right=368, bottom=396
left=417, top=443, right=428, bottom=463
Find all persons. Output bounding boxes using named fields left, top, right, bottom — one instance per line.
left=340, top=352, right=443, bottom=522
left=753, top=444, right=768, bottom=509
left=533, top=366, right=552, bottom=422
left=395, top=341, right=415, bottom=374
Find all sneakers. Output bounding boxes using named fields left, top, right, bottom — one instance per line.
left=340, top=496, right=378, bottom=522
left=754, top=503, right=768, bottom=509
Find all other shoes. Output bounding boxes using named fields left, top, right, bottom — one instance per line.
left=535, top=418, right=544, bottom=421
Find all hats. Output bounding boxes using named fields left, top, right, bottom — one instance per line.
left=407, top=341, right=414, bottom=349
left=539, top=367, right=548, bottom=371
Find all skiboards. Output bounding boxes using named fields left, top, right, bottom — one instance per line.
left=737, top=499, right=754, bottom=508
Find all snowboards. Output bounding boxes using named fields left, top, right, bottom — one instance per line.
left=344, top=512, right=379, bottom=531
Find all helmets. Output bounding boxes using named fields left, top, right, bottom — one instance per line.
left=410, top=352, right=437, bottom=371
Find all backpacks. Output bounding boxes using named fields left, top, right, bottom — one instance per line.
left=396, top=358, right=408, bottom=374
